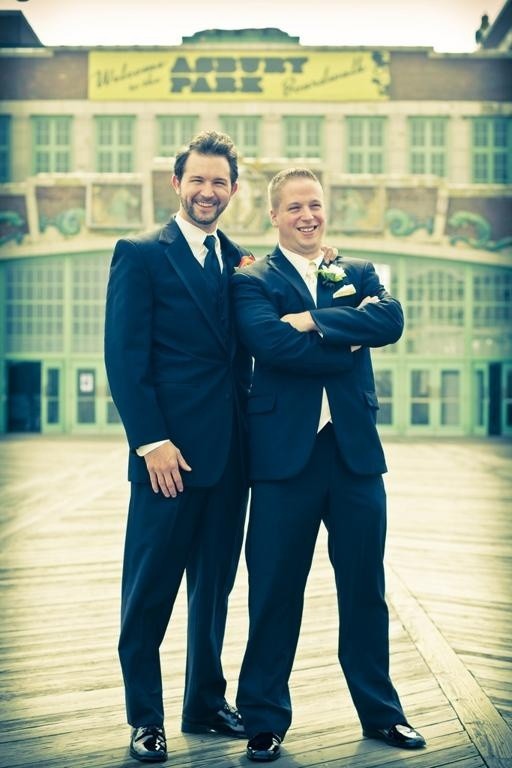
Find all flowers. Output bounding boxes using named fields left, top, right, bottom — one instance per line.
left=234, top=252, right=261, bottom=275
left=314, top=257, right=348, bottom=289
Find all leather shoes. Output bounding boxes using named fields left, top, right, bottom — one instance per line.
left=182, top=703, right=249, bottom=739
left=362, top=721, right=426, bottom=749
left=131, top=726, right=169, bottom=763
left=246, top=731, right=279, bottom=763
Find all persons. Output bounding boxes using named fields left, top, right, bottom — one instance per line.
left=102, top=127, right=342, bottom=763
left=226, top=166, right=427, bottom=762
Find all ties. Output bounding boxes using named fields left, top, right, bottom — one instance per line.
left=305, top=262, right=318, bottom=303
left=204, top=236, right=222, bottom=326
